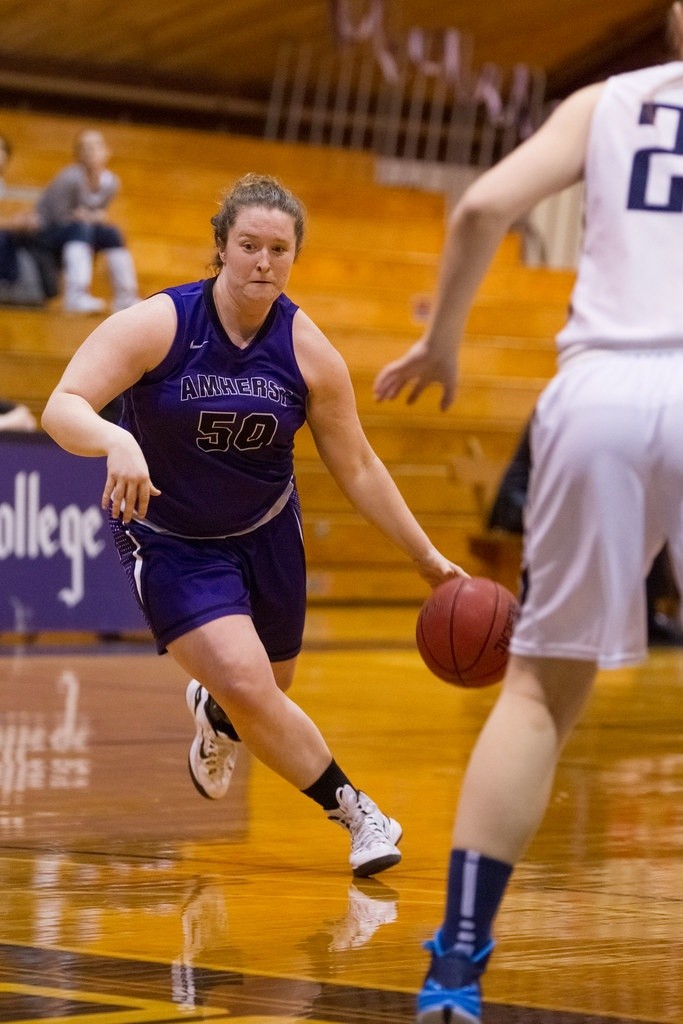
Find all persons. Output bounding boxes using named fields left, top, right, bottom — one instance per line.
left=372, top=0, right=682, bottom=1024
left=38, top=174, right=473, bottom=874
left=36, top=130, right=147, bottom=314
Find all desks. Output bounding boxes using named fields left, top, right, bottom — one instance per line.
left=0, top=414, right=150, bottom=658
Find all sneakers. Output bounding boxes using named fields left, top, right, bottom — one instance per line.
left=180, top=889, right=245, bottom=1008
left=185, top=676, right=238, bottom=799
left=417, top=930, right=494, bottom=1024
left=322, top=786, right=401, bottom=876
left=328, top=878, right=399, bottom=951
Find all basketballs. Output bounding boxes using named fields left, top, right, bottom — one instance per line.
left=415, top=576, right=522, bottom=689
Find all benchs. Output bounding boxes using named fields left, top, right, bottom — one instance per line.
left=0, top=112, right=569, bottom=611
